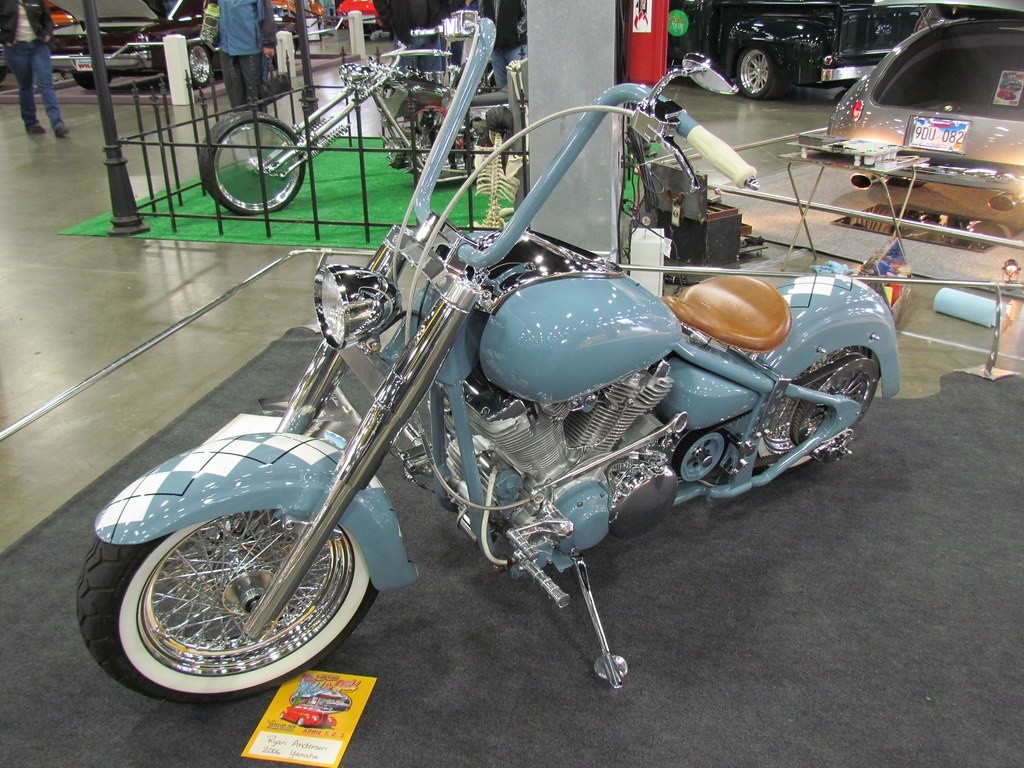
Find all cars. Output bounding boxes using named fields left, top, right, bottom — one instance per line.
left=47, top=2, right=309, bottom=91
left=336, top=1, right=394, bottom=44
left=370, top=0, right=926, bottom=101
left=774, top=18, right=1023, bottom=228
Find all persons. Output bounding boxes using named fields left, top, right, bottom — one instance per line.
left=202, top=0, right=277, bottom=111
left=472, top=0, right=527, bottom=90
left=0, top=0, right=69, bottom=138
left=372, top=0, right=464, bottom=84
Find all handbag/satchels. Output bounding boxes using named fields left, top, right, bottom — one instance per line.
left=201, top=2, right=221, bottom=45
left=257, top=55, right=292, bottom=105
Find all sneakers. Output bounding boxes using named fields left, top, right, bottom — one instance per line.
left=55, top=128, right=68, bottom=137
left=25, top=123, right=45, bottom=135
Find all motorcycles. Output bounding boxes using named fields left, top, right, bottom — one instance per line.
left=198, top=29, right=532, bottom=217
left=74, top=10, right=902, bottom=705
